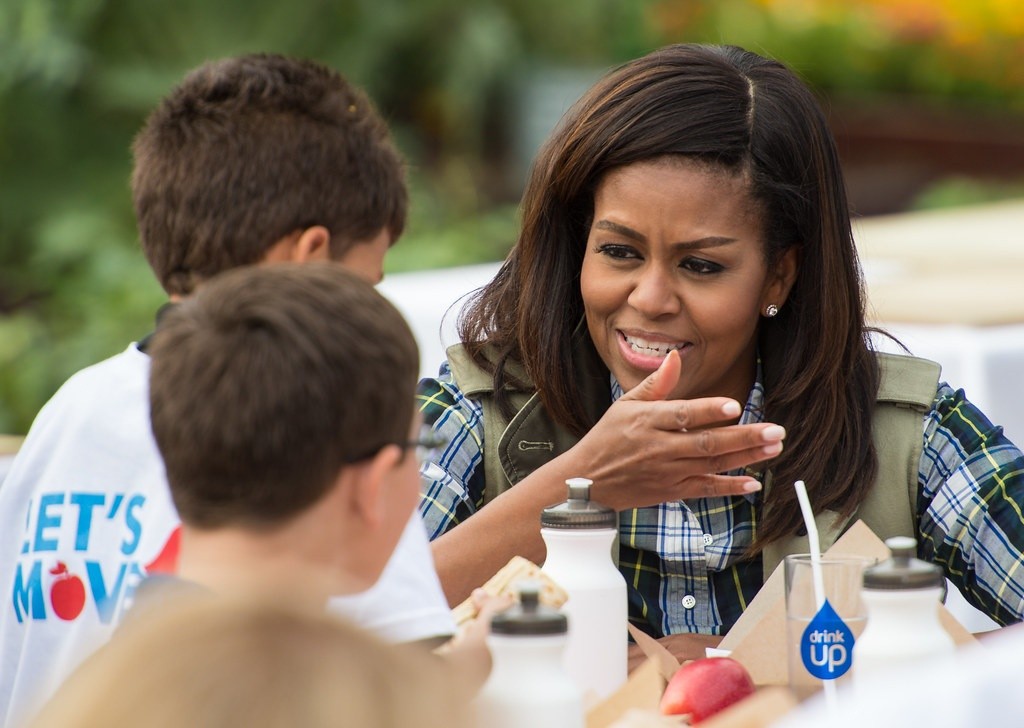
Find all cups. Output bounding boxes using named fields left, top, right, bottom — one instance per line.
left=784, top=554, right=880, bottom=702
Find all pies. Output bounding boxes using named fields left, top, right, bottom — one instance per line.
left=453, top=554, right=568, bottom=628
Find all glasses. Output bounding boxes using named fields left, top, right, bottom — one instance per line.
left=343, top=422, right=449, bottom=464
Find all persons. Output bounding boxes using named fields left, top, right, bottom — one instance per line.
left=417, top=42, right=1023, bottom=628
left=1, top=50, right=461, bottom=728
left=30, top=264, right=479, bottom=727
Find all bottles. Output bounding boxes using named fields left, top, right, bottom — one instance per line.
left=538, top=476, right=628, bottom=713
left=851, top=541, right=955, bottom=701
left=475, top=588, right=583, bottom=728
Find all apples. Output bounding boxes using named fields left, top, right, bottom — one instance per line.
left=661, top=656, right=755, bottom=726
